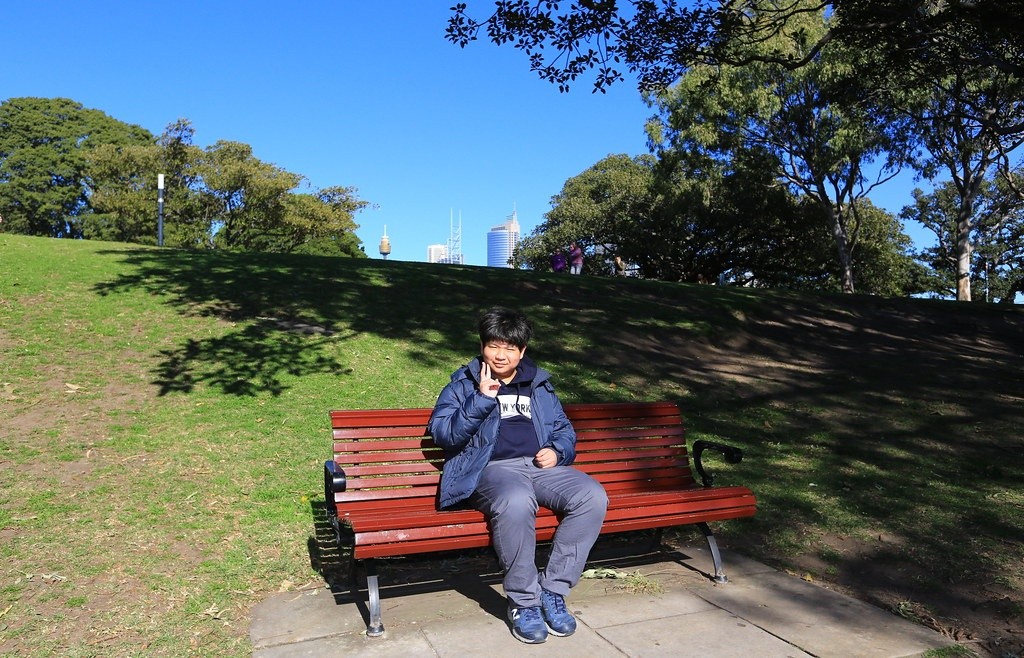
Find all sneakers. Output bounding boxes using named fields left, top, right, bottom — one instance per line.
left=538, top=582, right=578, bottom=637
left=507, top=604, right=549, bottom=644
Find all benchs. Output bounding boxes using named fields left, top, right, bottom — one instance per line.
left=325, top=401, right=755, bottom=637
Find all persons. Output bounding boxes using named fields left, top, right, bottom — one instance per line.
left=550, top=247, right=566, bottom=272
left=567, top=241, right=583, bottom=275
left=615, top=256, right=626, bottom=276
left=685, top=271, right=697, bottom=283
left=697, top=273, right=708, bottom=284
left=428, top=307, right=608, bottom=644
